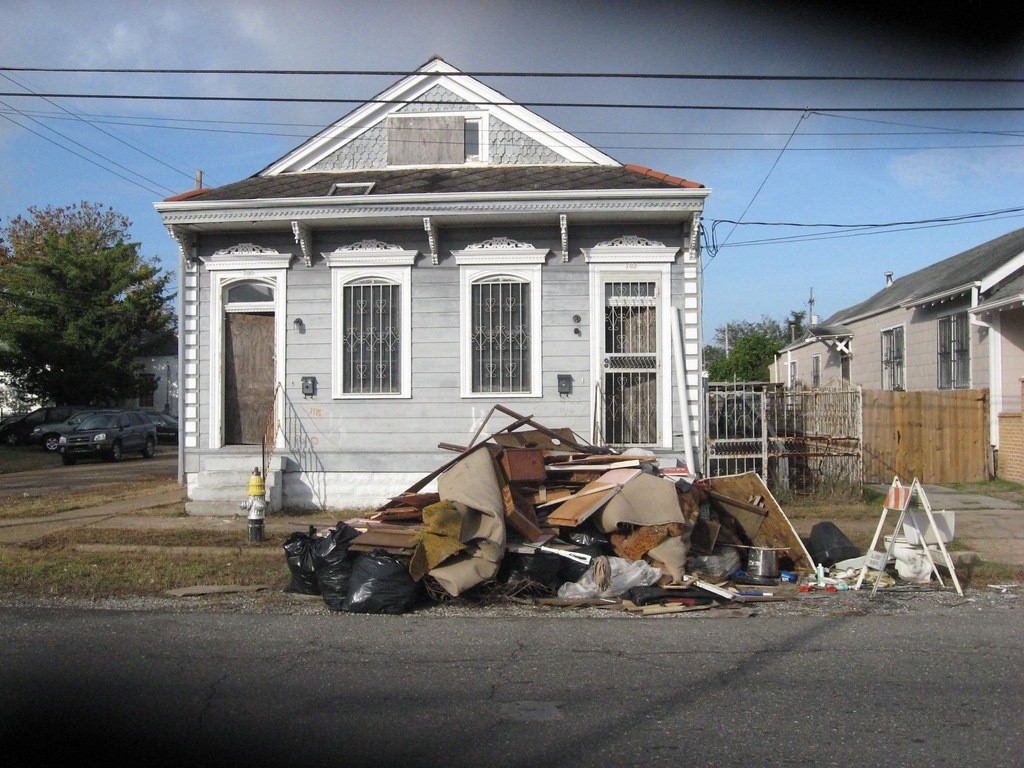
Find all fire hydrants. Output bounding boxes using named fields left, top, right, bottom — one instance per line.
left=239, top=466, right=271, bottom=542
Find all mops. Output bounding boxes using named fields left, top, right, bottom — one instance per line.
left=507, top=540, right=612, bottom=591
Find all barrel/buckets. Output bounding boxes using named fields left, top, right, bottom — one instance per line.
left=746, top=547, right=780, bottom=584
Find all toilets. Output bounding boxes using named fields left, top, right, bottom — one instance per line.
left=884, top=511, right=955, bottom=583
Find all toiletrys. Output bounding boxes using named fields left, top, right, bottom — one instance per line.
left=779, top=562, right=857, bottom=593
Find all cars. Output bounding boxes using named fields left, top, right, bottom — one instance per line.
left=147, top=412, right=178, bottom=444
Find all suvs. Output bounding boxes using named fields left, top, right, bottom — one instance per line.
left=30, top=408, right=126, bottom=452
left=0, top=407, right=85, bottom=446
left=58, top=408, right=157, bottom=465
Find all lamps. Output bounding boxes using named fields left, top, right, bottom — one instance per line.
left=293, top=319, right=303, bottom=329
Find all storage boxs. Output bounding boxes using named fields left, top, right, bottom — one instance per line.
left=498, top=447, right=545, bottom=482
left=501, top=482, right=544, bottom=542
